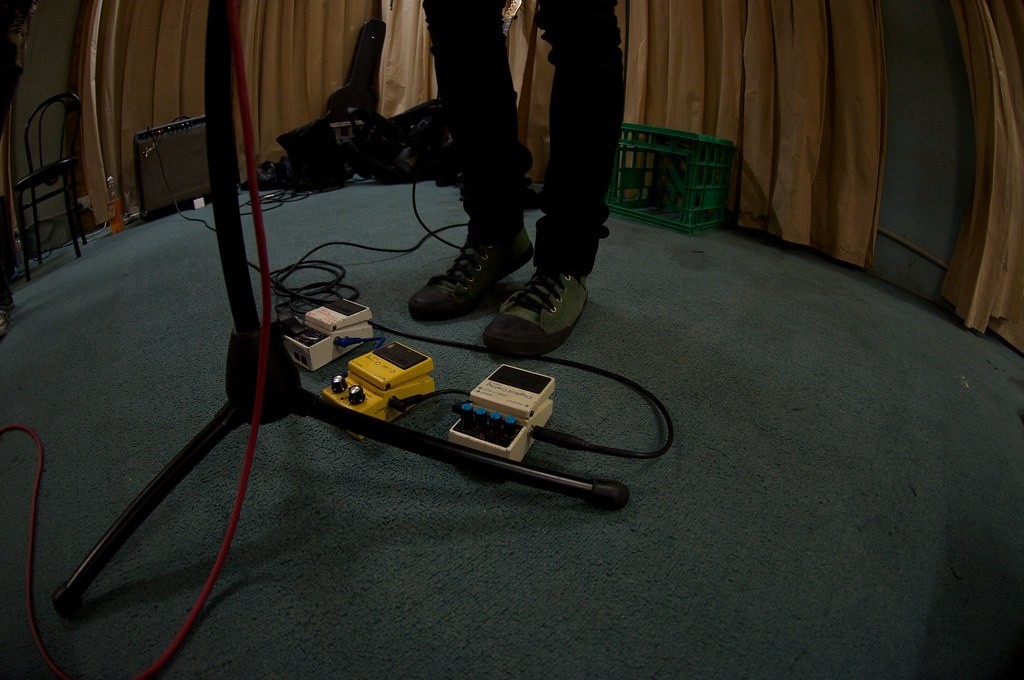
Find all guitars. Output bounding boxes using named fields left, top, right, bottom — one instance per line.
left=502, top=0, right=523, bottom=36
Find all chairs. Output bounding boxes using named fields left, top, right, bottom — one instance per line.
left=17, top=92, right=90, bottom=282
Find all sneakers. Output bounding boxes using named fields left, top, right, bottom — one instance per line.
left=408, top=225, right=534, bottom=322
left=482, top=269, right=588, bottom=354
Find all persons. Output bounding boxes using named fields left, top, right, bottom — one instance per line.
left=407, top=0, right=627, bottom=363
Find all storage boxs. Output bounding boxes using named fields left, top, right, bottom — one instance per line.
left=606, top=121, right=735, bottom=236
